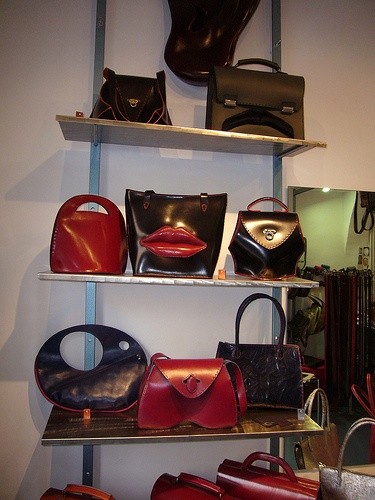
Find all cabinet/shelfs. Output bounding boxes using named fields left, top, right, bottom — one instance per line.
left=40, top=110, right=327, bottom=447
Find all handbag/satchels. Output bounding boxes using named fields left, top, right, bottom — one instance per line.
left=39, top=483, right=116, bottom=500
left=89, top=67, right=172, bottom=125
left=205, top=58, right=306, bottom=140
left=352, top=374, right=375, bottom=462
left=49, top=195, right=128, bottom=276
left=150, top=472, right=224, bottom=500
left=33, top=325, right=146, bottom=412
left=318, top=415, right=375, bottom=500
left=216, top=451, right=321, bottom=500
left=216, top=293, right=303, bottom=411
left=291, top=389, right=343, bottom=470
left=287, top=186, right=375, bottom=275
left=125, top=189, right=228, bottom=279
left=229, top=196, right=305, bottom=281
left=135, top=354, right=248, bottom=429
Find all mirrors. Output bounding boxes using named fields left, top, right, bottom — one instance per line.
left=285, top=186, right=375, bottom=467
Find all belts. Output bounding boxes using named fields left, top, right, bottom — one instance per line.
left=326, top=265, right=374, bottom=413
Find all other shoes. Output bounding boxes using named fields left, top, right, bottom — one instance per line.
left=292, top=301, right=327, bottom=353
